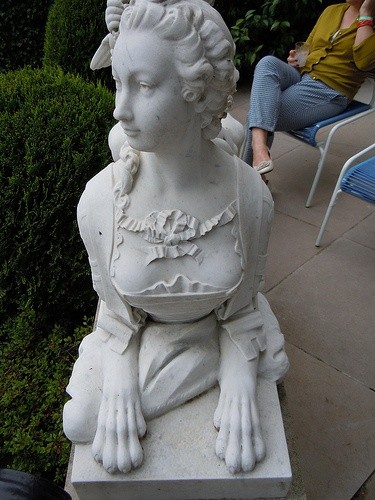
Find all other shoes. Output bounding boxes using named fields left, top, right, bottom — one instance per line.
left=251, top=161, right=273, bottom=176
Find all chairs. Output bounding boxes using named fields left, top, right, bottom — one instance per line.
left=272, top=71, right=375, bottom=207
left=314, top=142, right=375, bottom=253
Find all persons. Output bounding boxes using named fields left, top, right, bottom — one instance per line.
left=61, top=0, right=292, bottom=473
left=241, top=0, right=375, bottom=184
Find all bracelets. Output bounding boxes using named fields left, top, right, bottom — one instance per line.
left=358, top=16, right=374, bottom=20
left=357, top=20, right=374, bottom=28
